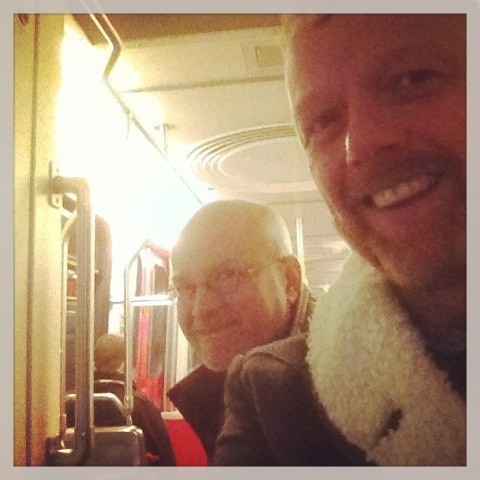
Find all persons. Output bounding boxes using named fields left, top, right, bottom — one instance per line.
left=92, top=333, right=176, bottom=467
left=211, top=15, right=466, bottom=467
left=165, top=197, right=321, bottom=466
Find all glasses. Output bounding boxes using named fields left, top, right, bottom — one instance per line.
left=172, top=261, right=267, bottom=301
